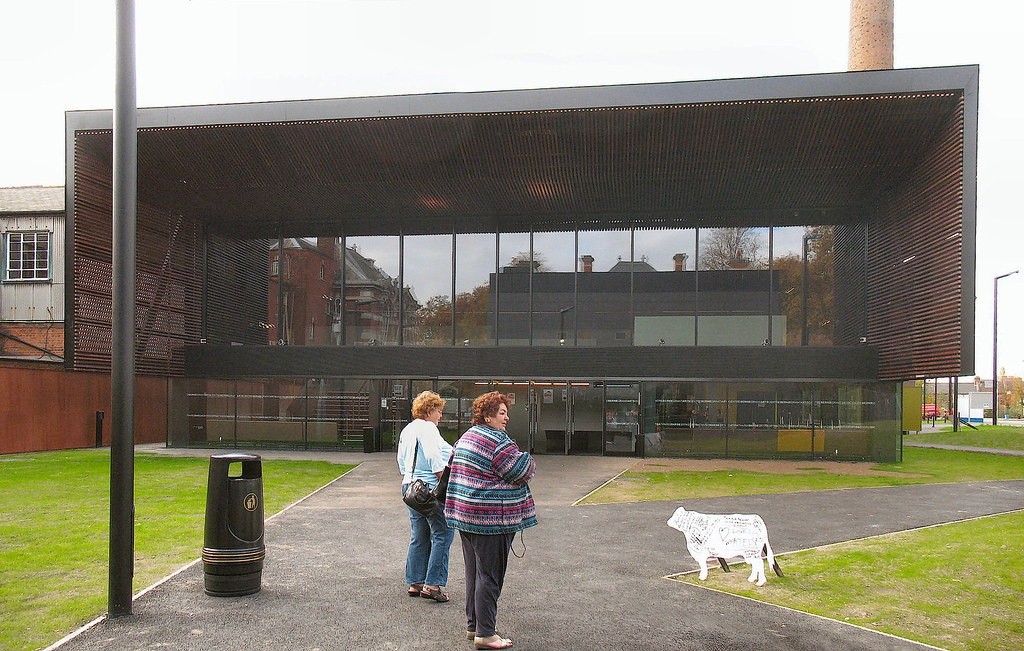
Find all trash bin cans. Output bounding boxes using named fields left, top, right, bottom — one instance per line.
left=201, top=452, right=265, bottom=598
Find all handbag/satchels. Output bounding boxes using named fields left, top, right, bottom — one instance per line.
left=431, top=453, right=451, bottom=504
left=402, top=479, right=438, bottom=517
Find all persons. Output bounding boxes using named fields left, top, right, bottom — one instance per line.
left=396, top=390, right=453, bottom=602
left=442, top=390, right=538, bottom=649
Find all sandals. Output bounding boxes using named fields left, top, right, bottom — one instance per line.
left=408, top=584, right=424, bottom=596
left=466, top=631, right=478, bottom=639
left=420, top=585, right=449, bottom=602
left=473, top=633, right=512, bottom=649
